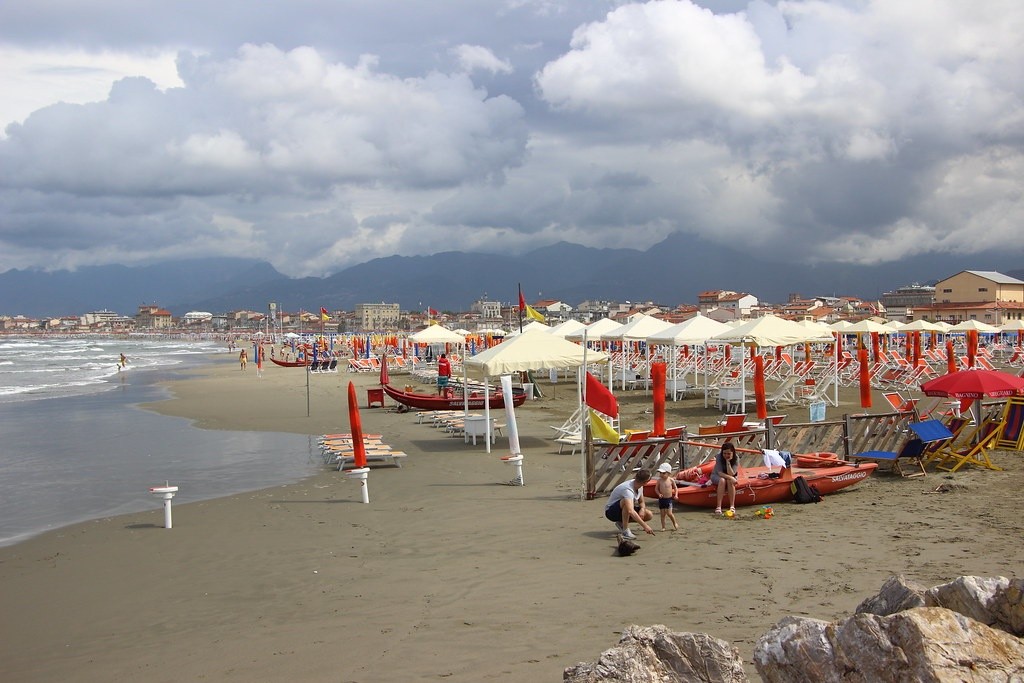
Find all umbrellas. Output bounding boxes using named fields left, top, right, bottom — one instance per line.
left=920, top=370, right=1024, bottom=461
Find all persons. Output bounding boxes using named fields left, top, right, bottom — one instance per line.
left=228, top=341, right=306, bottom=362
left=240, top=349, right=247, bottom=371
left=117, top=364, right=121, bottom=370
left=120, top=353, right=125, bottom=366
left=438, top=354, right=451, bottom=395
left=605, top=470, right=656, bottom=540
left=655, top=463, right=678, bottom=532
left=710, top=443, right=739, bottom=514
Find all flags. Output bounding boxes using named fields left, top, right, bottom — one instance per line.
left=519, top=292, right=525, bottom=311
left=322, top=307, right=330, bottom=321
left=526, top=305, right=544, bottom=323
left=430, top=308, right=438, bottom=326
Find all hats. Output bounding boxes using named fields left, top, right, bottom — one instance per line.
left=657, top=462, right=672, bottom=473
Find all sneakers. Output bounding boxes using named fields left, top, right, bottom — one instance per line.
left=621, top=528, right=637, bottom=540
left=615, top=522, right=623, bottom=531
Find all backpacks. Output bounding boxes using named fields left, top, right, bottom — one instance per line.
left=791, top=476, right=819, bottom=503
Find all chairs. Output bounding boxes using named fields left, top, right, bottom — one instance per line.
left=536, top=338, right=1024, bottom=479
left=307, top=354, right=497, bottom=397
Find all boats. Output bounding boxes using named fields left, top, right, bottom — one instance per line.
left=382, top=384, right=527, bottom=410
left=270, top=358, right=322, bottom=367
left=643, top=463, right=878, bottom=507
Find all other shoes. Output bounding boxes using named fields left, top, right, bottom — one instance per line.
left=715, top=507, right=722, bottom=515
left=730, top=506, right=735, bottom=513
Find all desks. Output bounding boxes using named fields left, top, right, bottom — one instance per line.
left=664, top=378, right=687, bottom=400
left=466, top=415, right=495, bottom=446
left=616, top=370, right=636, bottom=390
left=718, top=386, right=742, bottom=412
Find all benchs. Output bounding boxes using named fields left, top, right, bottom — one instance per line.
left=414, top=409, right=505, bottom=438
left=315, top=432, right=408, bottom=472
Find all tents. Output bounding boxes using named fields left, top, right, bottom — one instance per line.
left=646, top=315, right=735, bottom=401
left=463, top=329, right=611, bottom=453
left=796, top=319, right=1024, bottom=355
left=503, top=317, right=625, bottom=382
left=600, top=315, right=676, bottom=390
left=408, top=324, right=507, bottom=371
left=704, top=314, right=839, bottom=407
